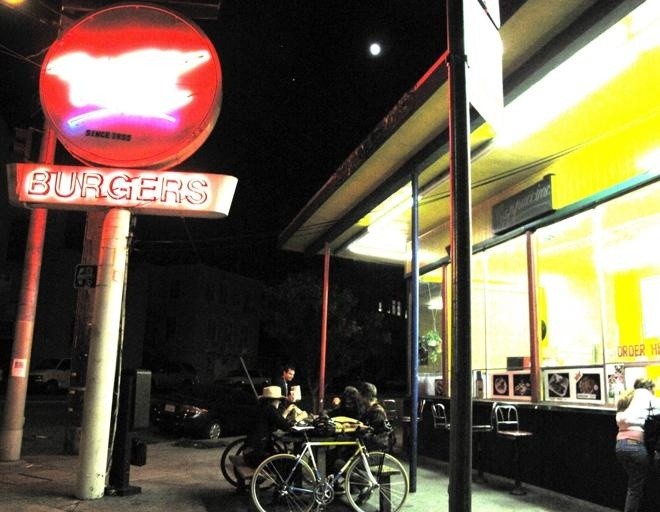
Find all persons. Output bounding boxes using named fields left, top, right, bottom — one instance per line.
left=230, top=364, right=397, bottom=500
left=614, top=379, right=660, bottom=511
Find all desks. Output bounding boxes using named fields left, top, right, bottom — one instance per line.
left=274, top=424, right=378, bottom=511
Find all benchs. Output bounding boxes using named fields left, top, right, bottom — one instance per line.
left=229, top=453, right=267, bottom=511
left=325, top=445, right=402, bottom=512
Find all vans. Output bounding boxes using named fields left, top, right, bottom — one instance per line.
left=380, top=398, right=402, bottom=421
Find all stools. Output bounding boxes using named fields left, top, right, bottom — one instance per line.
left=401, top=399, right=426, bottom=459
left=493, top=405, right=533, bottom=496
left=431, top=404, right=453, bottom=431
left=472, top=399, right=500, bottom=484
left=383, top=398, right=398, bottom=455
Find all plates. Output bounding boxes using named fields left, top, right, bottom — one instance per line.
left=495, top=378, right=508, bottom=394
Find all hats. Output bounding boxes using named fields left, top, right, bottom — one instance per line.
left=258, top=385, right=288, bottom=400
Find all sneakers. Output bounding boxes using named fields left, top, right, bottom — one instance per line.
left=356, top=490, right=374, bottom=506
left=335, top=487, right=345, bottom=495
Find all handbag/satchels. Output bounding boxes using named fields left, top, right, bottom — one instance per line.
left=306, top=417, right=336, bottom=437
left=291, top=385, right=301, bottom=400
left=644, top=399, right=660, bottom=452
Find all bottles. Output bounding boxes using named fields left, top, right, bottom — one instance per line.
left=476, top=371, right=484, bottom=400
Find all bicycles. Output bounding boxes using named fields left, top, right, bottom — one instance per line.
left=219, top=422, right=413, bottom=512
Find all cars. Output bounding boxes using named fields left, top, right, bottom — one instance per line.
left=147, top=382, right=269, bottom=440
left=219, top=367, right=272, bottom=387
left=27, top=354, right=72, bottom=395
